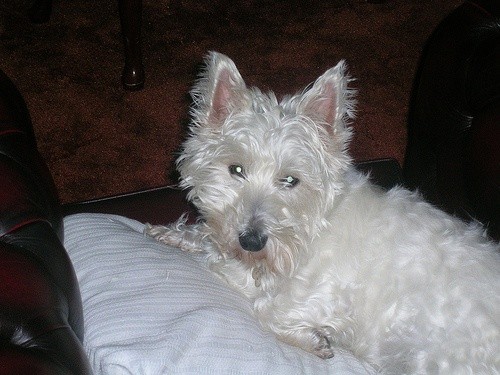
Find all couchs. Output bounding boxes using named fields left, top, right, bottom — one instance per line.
left=0, top=0, right=500, bottom=375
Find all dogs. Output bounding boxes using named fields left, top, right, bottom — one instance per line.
left=145, top=51, right=500, bottom=374
